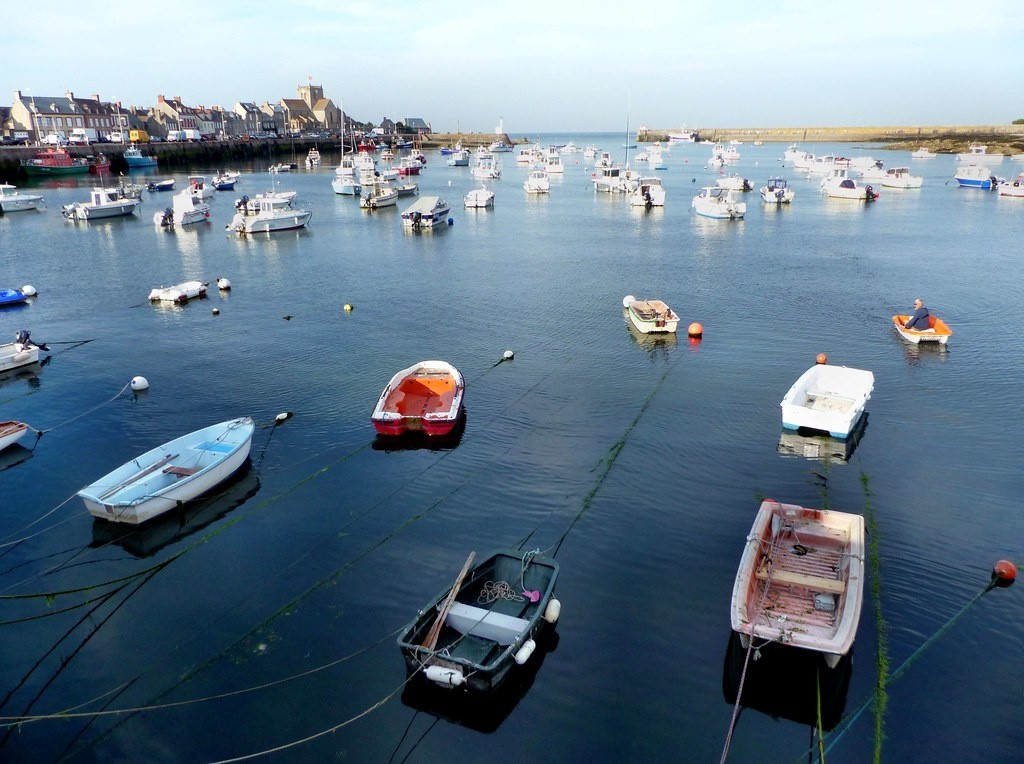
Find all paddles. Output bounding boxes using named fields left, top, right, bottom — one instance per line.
left=758, top=510, right=787, bottom=573
left=421, top=549, right=476, bottom=652
left=97, top=451, right=179, bottom=502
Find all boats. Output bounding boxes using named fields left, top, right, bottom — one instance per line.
left=779, top=364, right=876, bottom=440
left=699, top=139, right=1024, bottom=200
left=463, top=184, right=495, bottom=208
left=731, top=498, right=866, bottom=670
left=148, top=280, right=208, bottom=303
left=0, top=340, right=40, bottom=374
left=631, top=176, right=666, bottom=207
left=19, top=140, right=90, bottom=179
left=370, top=359, right=466, bottom=439
left=893, top=314, right=954, bottom=345
left=0, top=93, right=697, bottom=235
left=0, top=419, right=29, bottom=452
left=88, top=151, right=112, bottom=173
left=397, top=548, right=561, bottom=695
left=401, top=195, right=449, bottom=227
left=628, top=299, right=680, bottom=334
left=117, top=142, right=158, bottom=168
left=76, top=415, right=255, bottom=529
left=0, top=182, right=44, bottom=212
left=0, top=287, right=28, bottom=307
left=761, top=176, right=794, bottom=204
left=693, top=187, right=747, bottom=219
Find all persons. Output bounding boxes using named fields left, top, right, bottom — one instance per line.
left=905, top=299, right=929, bottom=330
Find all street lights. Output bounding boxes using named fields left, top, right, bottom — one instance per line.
left=173, top=102, right=182, bottom=141
left=282, top=110, right=287, bottom=138
left=253, top=108, right=259, bottom=136
